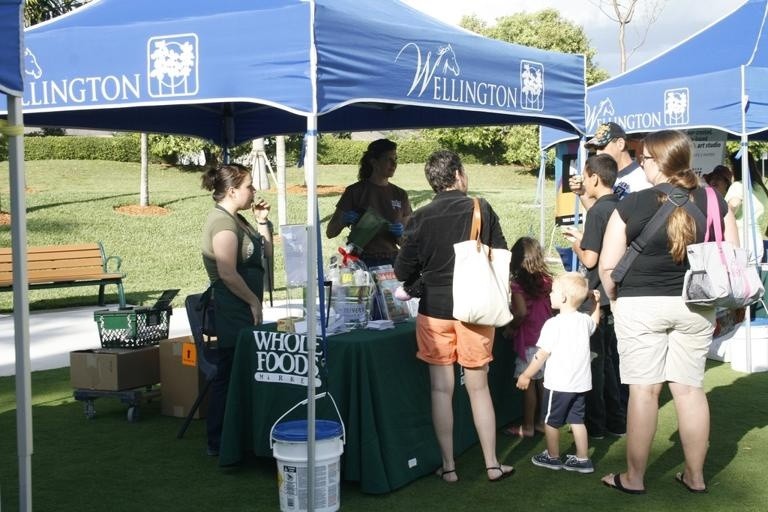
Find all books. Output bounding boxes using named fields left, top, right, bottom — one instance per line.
left=368, top=262, right=412, bottom=326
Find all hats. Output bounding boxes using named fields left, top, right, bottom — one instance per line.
left=584, top=122, right=626, bottom=147
left=702, top=165, right=733, bottom=185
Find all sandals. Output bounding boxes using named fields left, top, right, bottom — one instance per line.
left=485, top=463, right=515, bottom=482
left=437, top=467, right=458, bottom=483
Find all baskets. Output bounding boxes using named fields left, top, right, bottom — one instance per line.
left=94, top=307, right=170, bottom=348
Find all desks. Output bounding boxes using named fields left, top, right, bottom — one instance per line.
left=219, top=314, right=525, bottom=495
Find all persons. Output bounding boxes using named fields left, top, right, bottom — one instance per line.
left=393, top=150, right=516, bottom=484
left=199, top=161, right=274, bottom=455
left=562, top=153, right=629, bottom=440
left=597, top=129, right=741, bottom=495
left=568, top=122, right=653, bottom=228
left=325, top=138, right=414, bottom=321
left=515, top=272, right=602, bottom=474
left=502, top=236, right=559, bottom=439
left=701, top=164, right=733, bottom=199
left=703, top=150, right=768, bottom=320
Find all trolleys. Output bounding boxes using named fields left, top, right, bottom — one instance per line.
left=73, top=383, right=162, bottom=422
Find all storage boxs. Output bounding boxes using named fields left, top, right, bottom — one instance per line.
left=69, top=347, right=159, bottom=390
left=160, top=335, right=204, bottom=418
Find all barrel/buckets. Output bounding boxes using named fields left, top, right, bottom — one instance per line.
left=268, top=391, right=347, bottom=511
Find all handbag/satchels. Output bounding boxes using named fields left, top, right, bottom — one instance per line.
left=200, top=300, right=216, bottom=336
left=452, top=241, right=513, bottom=328
left=682, top=242, right=765, bottom=308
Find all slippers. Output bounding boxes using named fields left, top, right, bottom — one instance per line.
left=504, top=425, right=533, bottom=437
left=676, top=473, right=707, bottom=493
left=602, top=473, right=647, bottom=495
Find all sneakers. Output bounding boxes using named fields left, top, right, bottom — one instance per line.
left=531, top=450, right=563, bottom=470
left=566, top=454, right=594, bottom=474
left=608, top=423, right=627, bottom=434
left=588, top=426, right=605, bottom=440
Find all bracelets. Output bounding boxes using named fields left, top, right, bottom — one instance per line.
left=509, top=321, right=517, bottom=330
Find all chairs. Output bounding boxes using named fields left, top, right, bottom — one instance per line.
left=175, top=294, right=222, bottom=440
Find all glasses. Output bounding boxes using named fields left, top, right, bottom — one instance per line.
left=639, top=154, right=654, bottom=162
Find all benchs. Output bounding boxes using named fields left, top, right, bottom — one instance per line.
left=0, top=240, right=126, bottom=310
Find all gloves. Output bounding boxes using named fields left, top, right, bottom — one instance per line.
left=338, top=210, right=359, bottom=228
left=383, top=222, right=405, bottom=238
